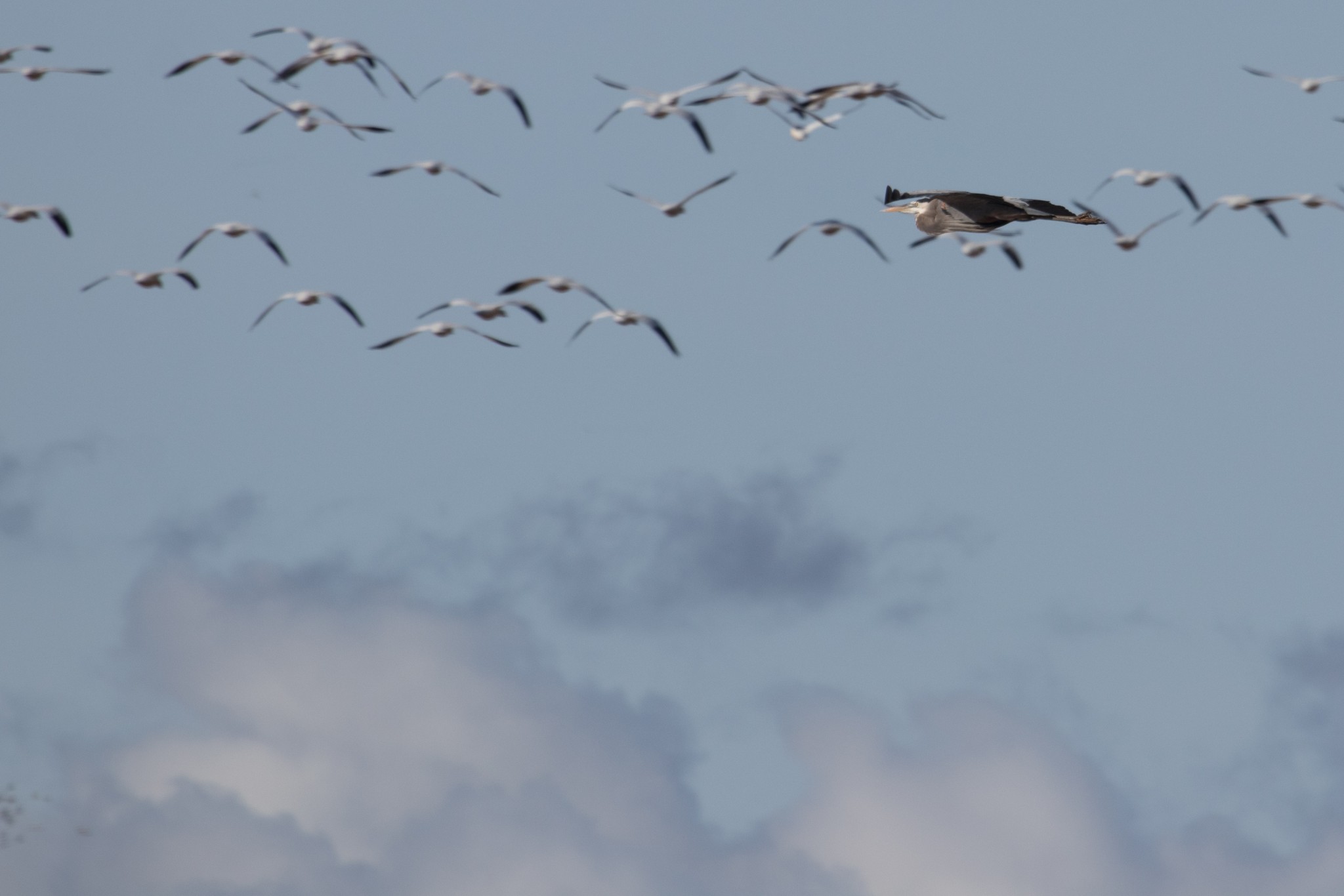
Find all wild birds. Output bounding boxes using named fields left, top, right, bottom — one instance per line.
left=178, top=223, right=288, bottom=267
left=1071, top=199, right=1184, bottom=249
left=0, top=201, right=72, bottom=239
left=79, top=268, right=200, bottom=295
left=588, top=67, right=944, bottom=155
left=161, top=25, right=533, bottom=199
left=1235, top=61, right=1344, bottom=95
left=0, top=44, right=52, bottom=65
left=0, top=62, right=110, bottom=81
left=768, top=220, right=889, bottom=263
left=1088, top=168, right=1202, bottom=212
left=568, top=309, right=681, bottom=356
left=607, top=171, right=736, bottom=217
left=910, top=232, right=1024, bottom=272
left=878, top=186, right=1108, bottom=233
left=248, top=290, right=364, bottom=332
left=424, top=298, right=547, bottom=326
left=490, top=276, right=617, bottom=314
left=1194, top=191, right=1344, bottom=239
left=369, top=323, right=521, bottom=352
